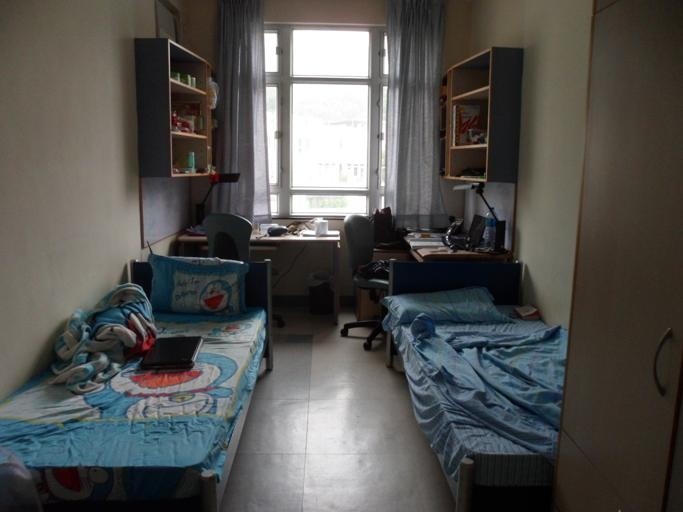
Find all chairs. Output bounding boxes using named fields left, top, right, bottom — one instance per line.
left=341, top=215, right=389, bottom=351
left=202, top=213, right=284, bottom=323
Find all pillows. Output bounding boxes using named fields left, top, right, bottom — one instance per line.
left=380, top=286, right=513, bottom=323
left=149, top=253, right=249, bottom=314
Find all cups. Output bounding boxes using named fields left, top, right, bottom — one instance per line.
left=406, top=226, right=416, bottom=237
left=467, top=127, right=482, bottom=144
left=419, top=226, right=432, bottom=238
left=259, top=223, right=277, bottom=235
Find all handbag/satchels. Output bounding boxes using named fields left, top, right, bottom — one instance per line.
left=371, top=207, right=392, bottom=242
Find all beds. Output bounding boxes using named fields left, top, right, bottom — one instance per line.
left=389, top=258, right=569, bottom=512
left=0, top=260, right=274, bottom=512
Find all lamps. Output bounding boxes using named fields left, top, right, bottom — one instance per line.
left=450, top=182, right=501, bottom=225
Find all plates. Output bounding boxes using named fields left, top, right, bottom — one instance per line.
left=302, top=230, right=339, bottom=236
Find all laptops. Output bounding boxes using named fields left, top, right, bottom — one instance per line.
left=443, top=215, right=488, bottom=250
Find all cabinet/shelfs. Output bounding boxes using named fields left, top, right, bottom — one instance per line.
left=134, top=38, right=213, bottom=177
left=439, top=46, right=523, bottom=184
left=354, top=248, right=409, bottom=321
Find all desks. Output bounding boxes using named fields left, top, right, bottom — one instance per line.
left=177, top=230, right=340, bottom=325
left=405, top=230, right=511, bottom=263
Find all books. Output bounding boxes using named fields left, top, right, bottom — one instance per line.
left=409, top=240, right=444, bottom=249
left=513, top=304, right=540, bottom=321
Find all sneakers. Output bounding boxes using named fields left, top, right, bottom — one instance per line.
left=358, top=260, right=390, bottom=279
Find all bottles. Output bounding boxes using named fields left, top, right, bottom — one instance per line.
left=179, top=104, right=195, bottom=133
left=484, top=208, right=497, bottom=249
left=186, top=151, right=195, bottom=169
left=196, top=109, right=204, bottom=129
left=252, top=220, right=259, bottom=234
left=171, top=72, right=196, bottom=87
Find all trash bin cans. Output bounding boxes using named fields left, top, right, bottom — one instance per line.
left=307, top=272, right=334, bottom=315
